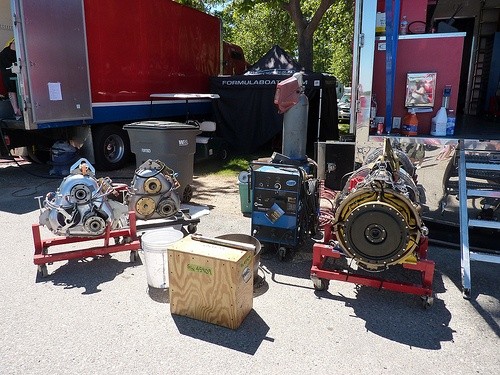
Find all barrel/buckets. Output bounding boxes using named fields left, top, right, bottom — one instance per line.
left=141, top=229, right=184, bottom=287
left=431, top=107, right=447, bottom=136
left=402, top=112, right=418, bottom=136
left=214, top=234, right=264, bottom=280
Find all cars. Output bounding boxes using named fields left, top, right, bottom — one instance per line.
left=337, top=87, right=351, bottom=123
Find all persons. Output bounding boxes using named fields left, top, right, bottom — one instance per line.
left=0, top=37, right=23, bottom=121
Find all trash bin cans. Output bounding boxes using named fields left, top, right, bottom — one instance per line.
left=122, top=119, right=200, bottom=204
left=324, top=142, right=355, bottom=191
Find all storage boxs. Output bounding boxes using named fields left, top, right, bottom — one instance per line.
left=167, top=234, right=256, bottom=331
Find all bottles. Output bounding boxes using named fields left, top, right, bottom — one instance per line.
left=446, top=110, right=455, bottom=136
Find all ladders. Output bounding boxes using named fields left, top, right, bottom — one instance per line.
left=458, top=139, right=500, bottom=299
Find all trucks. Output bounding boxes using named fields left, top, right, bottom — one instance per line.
left=351, top=1, right=500, bottom=257
left=0, top=0, right=252, bottom=171
